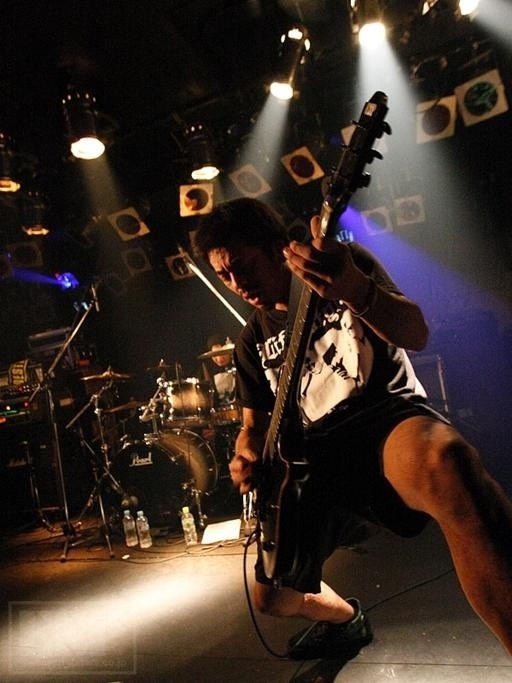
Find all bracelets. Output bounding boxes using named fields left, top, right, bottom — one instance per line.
left=347, top=275, right=380, bottom=320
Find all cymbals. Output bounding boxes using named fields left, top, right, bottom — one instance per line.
left=103, top=401, right=147, bottom=413
left=148, top=366, right=173, bottom=370
left=197, top=343, right=236, bottom=359
left=81, top=371, right=130, bottom=381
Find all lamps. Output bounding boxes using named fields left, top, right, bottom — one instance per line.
left=0, top=0, right=480, bottom=235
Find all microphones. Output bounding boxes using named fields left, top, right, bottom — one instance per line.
left=90, top=282, right=102, bottom=313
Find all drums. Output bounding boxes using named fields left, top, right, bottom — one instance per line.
left=110, top=428, right=218, bottom=528
left=163, top=377, right=215, bottom=428
left=213, top=405, right=241, bottom=427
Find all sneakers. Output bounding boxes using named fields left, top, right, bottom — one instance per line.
left=288, top=598, right=373, bottom=656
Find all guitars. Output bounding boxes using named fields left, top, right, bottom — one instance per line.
left=260, top=92, right=392, bottom=594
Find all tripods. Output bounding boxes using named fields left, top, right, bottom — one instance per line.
left=28, top=299, right=95, bottom=565
left=63, top=365, right=128, bottom=559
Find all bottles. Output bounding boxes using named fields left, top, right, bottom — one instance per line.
left=180, top=506, right=198, bottom=546
left=122, top=510, right=153, bottom=548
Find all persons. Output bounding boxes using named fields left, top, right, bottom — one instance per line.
left=192, top=198, right=512, bottom=661
left=196, top=330, right=241, bottom=401
